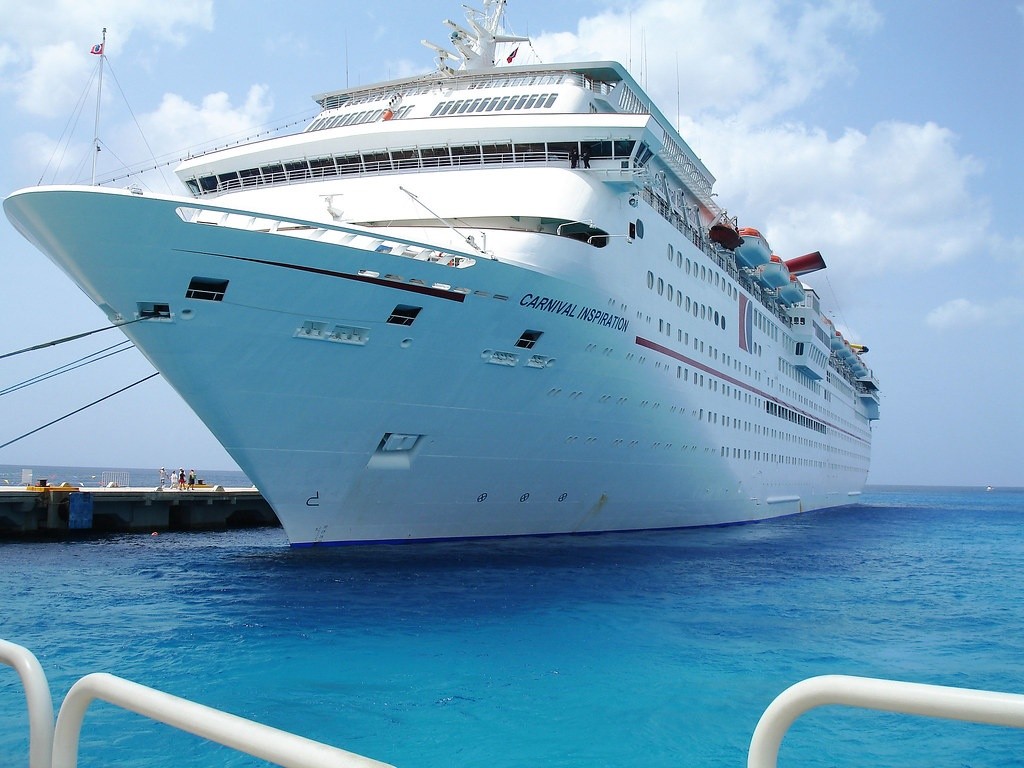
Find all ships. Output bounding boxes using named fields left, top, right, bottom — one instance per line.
left=1, top=1, right=879, bottom=549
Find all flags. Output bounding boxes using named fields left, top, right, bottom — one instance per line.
left=90, top=43, right=103, bottom=55
left=506, top=47, right=519, bottom=64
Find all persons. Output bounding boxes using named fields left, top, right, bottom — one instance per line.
left=169, top=470, right=178, bottom=489
left=159, top=466, right=169, bottom=487
left=582, top=147, right=591, bottom=169
left=177, top=467, right=196, bottom=491
left=569, top=147, right=580, bottom=168
